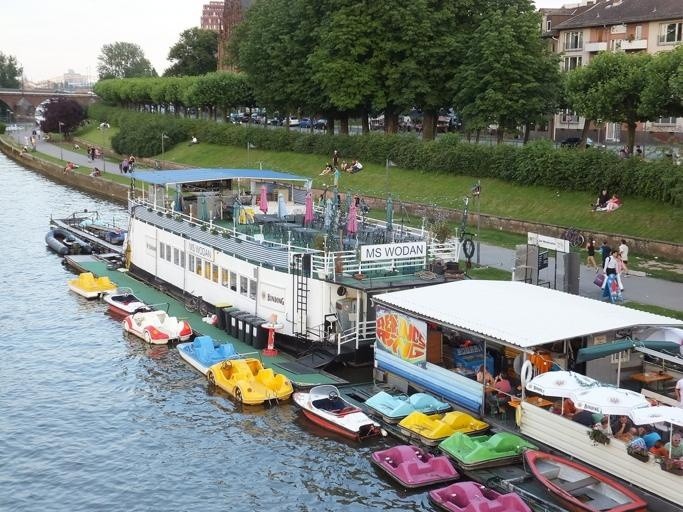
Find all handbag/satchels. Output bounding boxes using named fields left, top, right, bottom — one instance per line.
left=593, top=274, right=608, bottom=290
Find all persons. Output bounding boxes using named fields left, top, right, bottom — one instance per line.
left=188, top=136, right=198, bottom=146
left=452, top=364, right=683, bottom=473
left=585, top=236, right=631, bottom=298
left=62, top=146, right=135, bottom=178
left=595, top=189, right=609, bottom=208
left=318, top=149, right=365, bottom=189
left=23, top=127, right=40, bottom=152
left=605, top=195, right=622, bottom=213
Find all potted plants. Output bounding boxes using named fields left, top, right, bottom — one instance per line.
left=586, top=425, right=610, bottom=447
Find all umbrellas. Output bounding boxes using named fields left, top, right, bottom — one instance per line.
left=172, top=183, right=394, bottom=248
left=638, top=327, right=682, bottom=370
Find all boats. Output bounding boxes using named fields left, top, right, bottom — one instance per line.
left=397, top=410, right=490, bottom=445
left=66, top=161, right=465, bottom=389
left=369, top=277, right=683, bottom=510
left=524, top=447, right=649, bottom=512
left=372, top=447, right=457, bottom=488
left=440, top=430, right=541, bottom=471
left=46, top=229, right=91, bottom=254
left=48, top=208, right=125, bottom=256
left=429, top=481, right=534, bottom=512
left=365, top=391, right=451, bottom=424
left=292, top=384, right=384, bottom=441
left=69, top=271, right=294, bottom=403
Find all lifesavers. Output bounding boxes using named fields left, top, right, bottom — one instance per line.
left=521, top=361, right=533, bottom=385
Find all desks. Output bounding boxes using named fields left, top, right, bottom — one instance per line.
left=252, top=209, right=417, bottom=251
left=611, top=362, right=681, bottom=463
left=443, top=360, right=556, bottom=428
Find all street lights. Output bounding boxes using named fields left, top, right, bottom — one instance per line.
left=244, top=142, right=257, bottom=170
left=4, top=109, right=67, bottom=166
left=385, top=159, right=398, bottom=200
left=471, top=180, right=482, bottom=270
left=99, top=122, right=107, bottom=174
left=160, top=131, right=171, bottom=168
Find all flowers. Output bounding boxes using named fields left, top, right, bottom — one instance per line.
left=659, top=456, right=683, bottom=471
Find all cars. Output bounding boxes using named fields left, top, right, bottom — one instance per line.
left=561, top=137, right=591, bottom=147
left=220, top=110, right=455, bottom=134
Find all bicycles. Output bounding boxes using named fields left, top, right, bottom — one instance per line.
left=561, top=226, right=584, bottom=248
